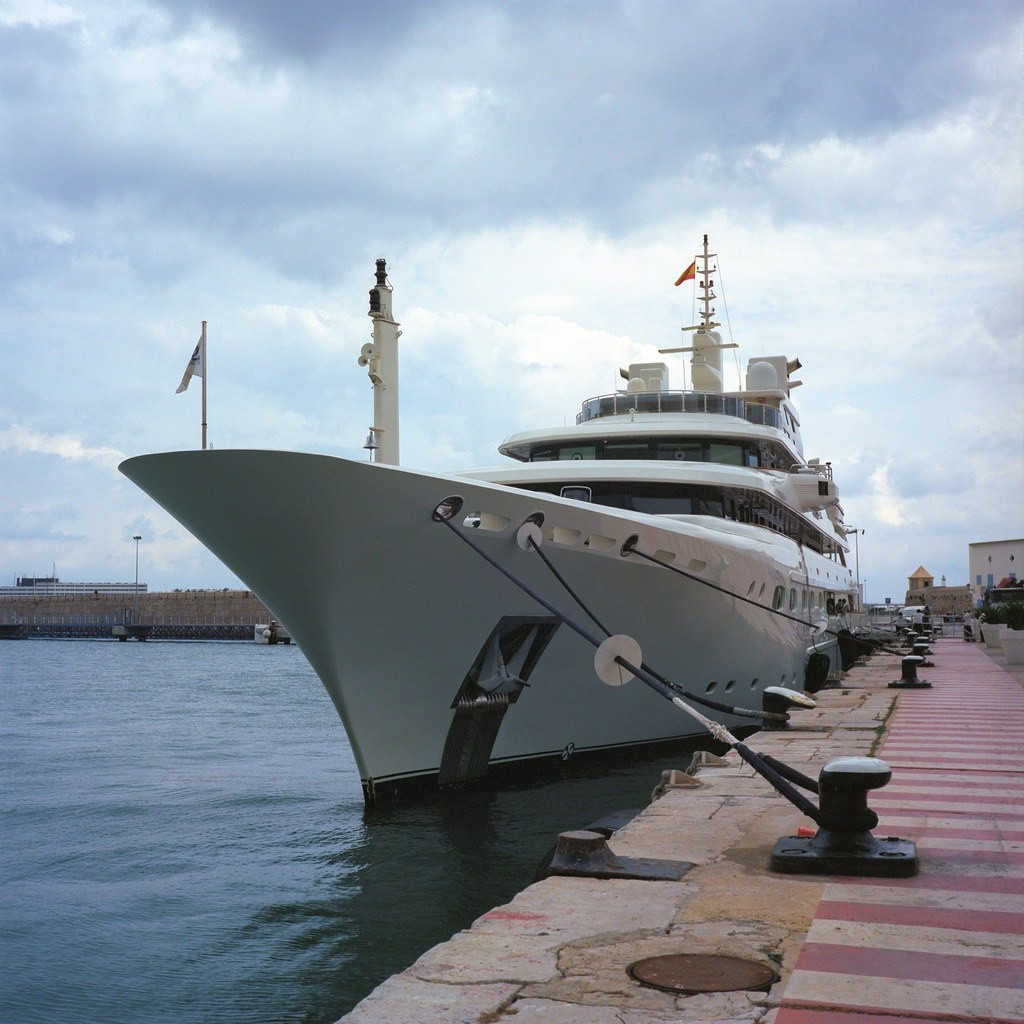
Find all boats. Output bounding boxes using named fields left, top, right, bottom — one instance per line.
left=117, top=230, right=867, bottom=804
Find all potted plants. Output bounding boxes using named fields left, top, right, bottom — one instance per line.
left=999, top=600, right=1024, bottom=665
left=980, top=605, right=1007, bottom=648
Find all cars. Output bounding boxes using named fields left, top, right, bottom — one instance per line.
left=894, top=606, right=938, bottom=635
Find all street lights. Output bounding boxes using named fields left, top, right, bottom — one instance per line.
left=132, top=536, right=143, bottom=596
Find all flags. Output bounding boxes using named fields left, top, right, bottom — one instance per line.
left=174, top=334, right=203, bottom=394
left=674, top=261, right=695, bottom=287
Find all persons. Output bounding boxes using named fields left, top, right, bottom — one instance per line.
left=892, top=612, right=910, bottom=633
left=962, top=610, right=976, bottom=643
left=910, top=609, right=925, bottom=636
left=826, top=598, right=849, bottom=615
